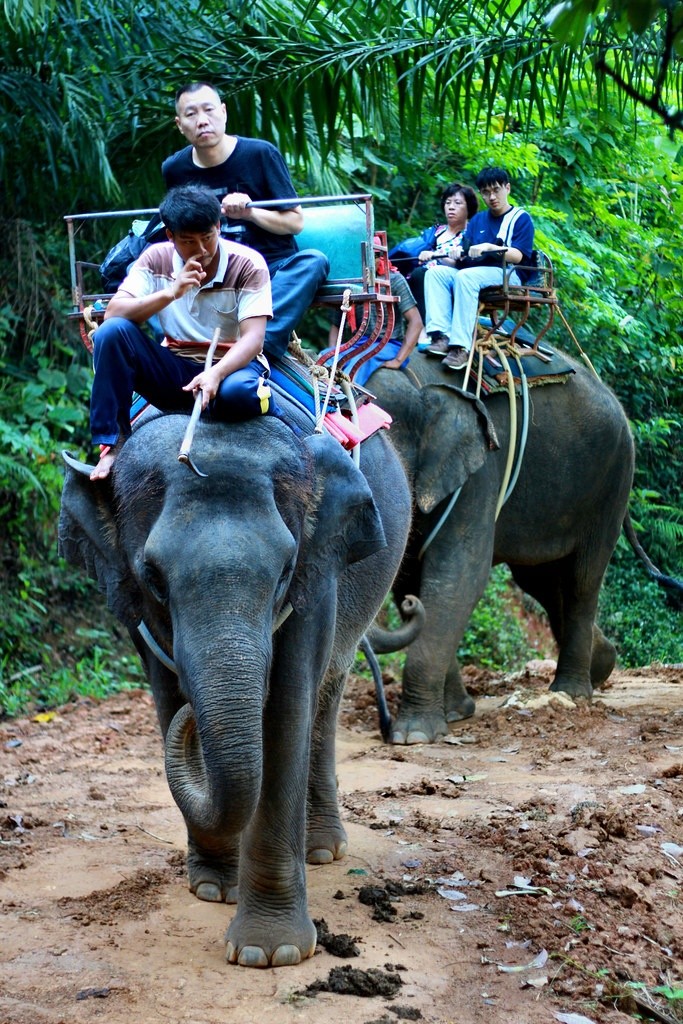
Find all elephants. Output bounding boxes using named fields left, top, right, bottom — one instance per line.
left=57, top=349, right=413, bottom=970
left=315, top=327, right=635, bottom=744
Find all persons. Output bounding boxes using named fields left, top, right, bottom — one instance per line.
left=89, top=185, right=275, bottom=480
left=161, top=81, right=330, bottom=360
left=405, top=167, right=534, bottom=369
left=318, top=236, right=424, bottom=398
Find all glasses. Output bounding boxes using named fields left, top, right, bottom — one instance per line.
left=482, top=187, right=502, bottom=197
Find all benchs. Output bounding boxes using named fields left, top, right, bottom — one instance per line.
left=474, top=233, right=558, bottom=365
left=62, top=196, right=401, bottom=377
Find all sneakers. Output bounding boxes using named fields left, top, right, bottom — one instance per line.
left=425, top=335, right=469, bottom=371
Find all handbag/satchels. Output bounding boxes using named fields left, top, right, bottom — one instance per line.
left=99, top=219, right=162, bottom=294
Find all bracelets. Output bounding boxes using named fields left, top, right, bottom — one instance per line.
left=172, top=284, right=177, bottom=301
